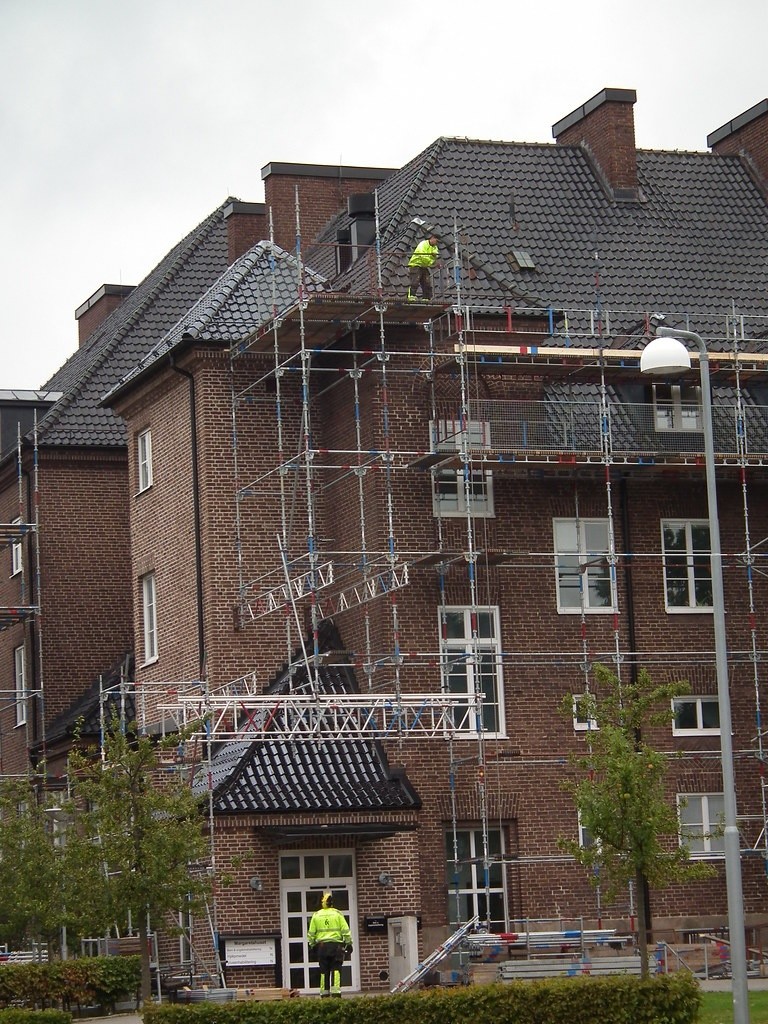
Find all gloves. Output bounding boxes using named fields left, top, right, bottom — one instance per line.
left=345, top=945, right=353, bottom=955
left=310, top=946, right=315, bottom=952
left=435, top=259, right=444, bottom=267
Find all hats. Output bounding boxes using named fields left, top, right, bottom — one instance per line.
left=322, top=893, right=333, bottom=904
left=435, top=234, right=442, bottom=242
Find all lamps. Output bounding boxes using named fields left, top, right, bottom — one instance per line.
left=250, top=877, right=263, bottom=890
left=379, top=872, right=396, bottom=890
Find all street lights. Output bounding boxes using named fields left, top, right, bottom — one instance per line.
left=640, top=326, right=749, bottom=1024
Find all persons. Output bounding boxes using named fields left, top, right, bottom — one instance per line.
left=309, top=893, right=353, bottom=997
left=408, top=233, right=444, bottom=302
